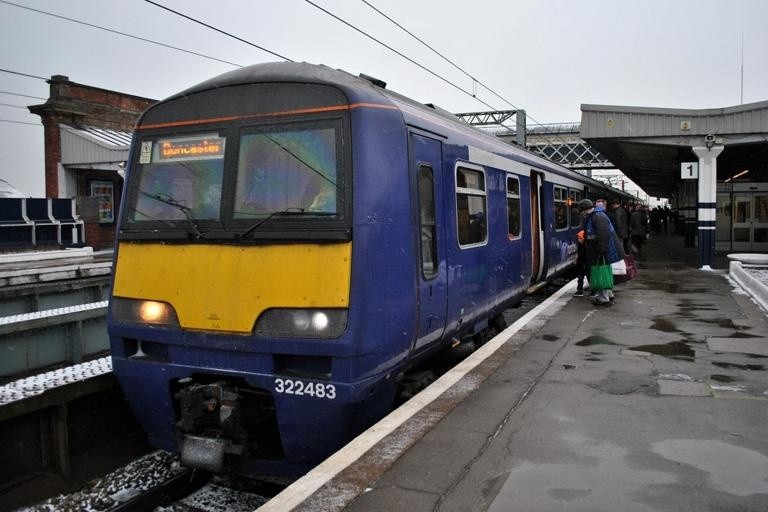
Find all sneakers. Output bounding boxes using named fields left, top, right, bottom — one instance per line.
left=573, top=292, right=616, bottom=306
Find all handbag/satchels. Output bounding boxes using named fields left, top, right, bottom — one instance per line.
left=612, top=254, right=637, bottom=285
left=590, top=264, right=613, bottom=290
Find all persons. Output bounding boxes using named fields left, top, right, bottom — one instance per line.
left=574, top=199, right=674, bottom=306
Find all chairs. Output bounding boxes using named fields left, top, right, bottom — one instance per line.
left=0, top=196, right=86, bottom=249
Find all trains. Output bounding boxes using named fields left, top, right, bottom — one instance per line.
left=105, top=61, right=641, bottom=479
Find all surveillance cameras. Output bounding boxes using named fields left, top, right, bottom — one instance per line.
left=705, top=135, right=715, bottom=142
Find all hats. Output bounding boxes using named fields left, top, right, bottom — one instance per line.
left=577, top=199, right=594, bottom=215
